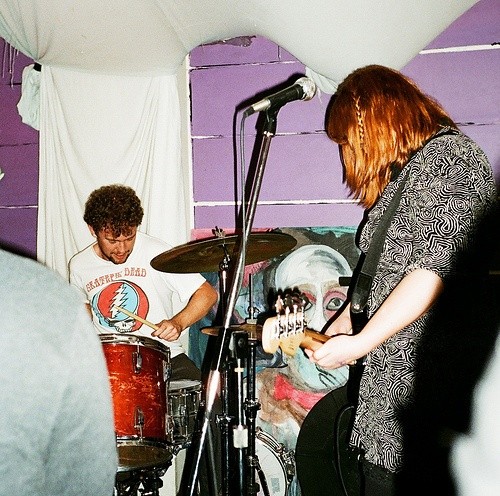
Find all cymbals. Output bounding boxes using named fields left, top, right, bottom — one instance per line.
left=150, top=233, right=297, bottom=274
left=202, top=324, right=263, bottom=340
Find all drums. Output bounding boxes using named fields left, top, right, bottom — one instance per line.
left=167, top=379, right=204, bottom=451
left=98, top=333, right=172, bottom=468
left=239, top=427, right=297, bottom=496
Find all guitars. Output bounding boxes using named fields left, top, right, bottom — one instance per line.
left=263, top=292, right=364, bottom=366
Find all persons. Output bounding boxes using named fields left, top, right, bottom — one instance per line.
left=0, top=249, right=119, bottom=495
left=313, top=64, right=500, bottom=495
left=68, top=184, right=218, bottom=382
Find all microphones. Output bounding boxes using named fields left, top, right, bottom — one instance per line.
left=243, top=77, right=317, bottom=118
